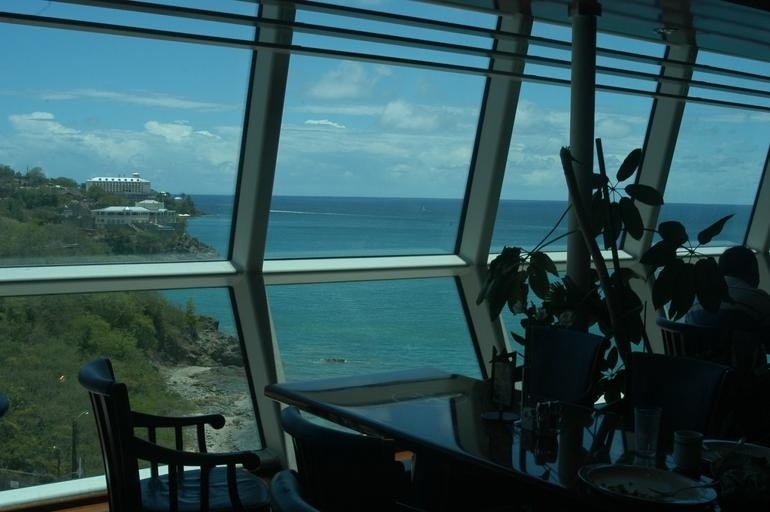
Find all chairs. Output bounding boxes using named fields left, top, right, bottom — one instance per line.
left=511, top=317, right=768, bottom=453
left=78, top=358, right=271, bottom=510
left=269, top=406, right=449, bottom=510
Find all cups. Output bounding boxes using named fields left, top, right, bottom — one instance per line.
left=672, top=430, right=709, bottom=466
left=632, top=405, right=664, bottom=459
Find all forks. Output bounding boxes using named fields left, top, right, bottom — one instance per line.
left=647, top=479, right=721, bottom=496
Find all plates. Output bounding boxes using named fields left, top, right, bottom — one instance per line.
left=576, top=462, right=718, bottom=506
left=698, top=438, right=770, bottom=466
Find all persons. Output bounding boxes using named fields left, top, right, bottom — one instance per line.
left=684, top=246, right=770, bottom=377
